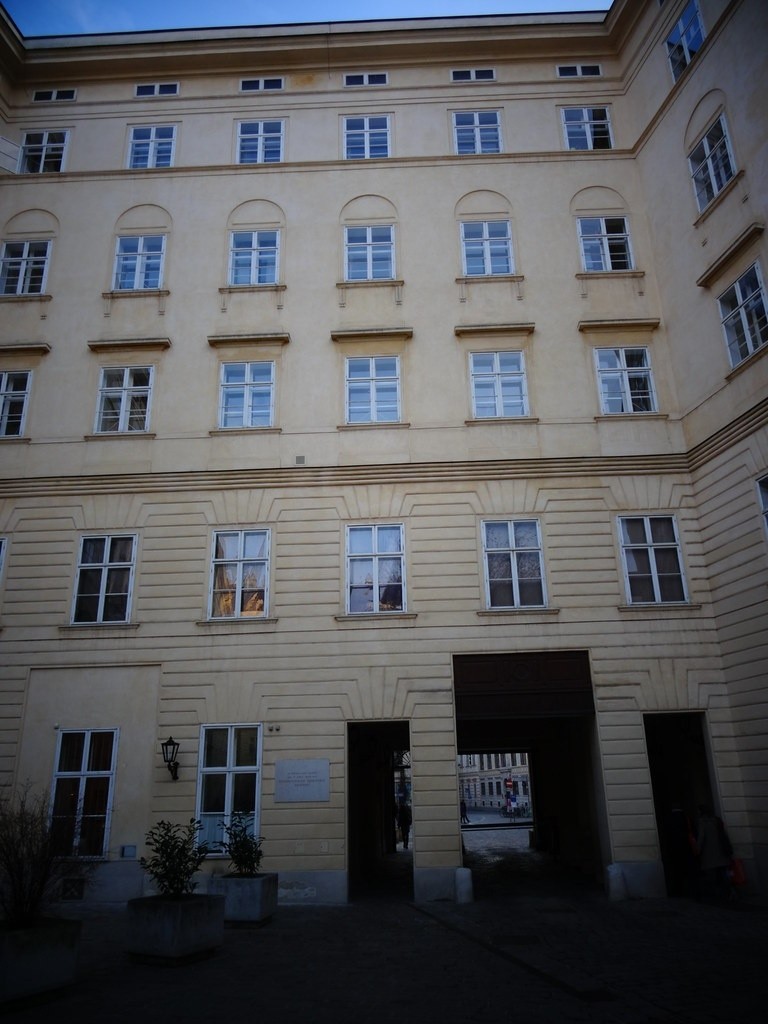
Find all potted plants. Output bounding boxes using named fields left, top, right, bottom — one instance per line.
left=127, top=818, right=225, bottom=969
left=206, top=807, right=279, bottom=930
left=0, top=774, right=81, bottom=1012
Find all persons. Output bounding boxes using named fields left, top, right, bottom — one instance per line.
left=460, top=799, right=470, bottom=824
left=693, top=804, right=736, bottom=907
left=397, top=799, right=412, bottom=849
left=663, top=802, right=698, bottom=884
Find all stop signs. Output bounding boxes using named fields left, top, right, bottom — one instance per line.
left=506, top=780, right=513, bottom=788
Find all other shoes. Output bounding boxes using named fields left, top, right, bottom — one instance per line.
left=728, top=888, right=736, bottom=903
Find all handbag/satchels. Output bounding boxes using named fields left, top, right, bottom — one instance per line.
left=730, top=859, right=747, bottom=884
left=717, top=816, right=734, bottom=855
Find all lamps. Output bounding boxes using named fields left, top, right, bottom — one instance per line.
left=161, top=736, right=182, bottom=784
